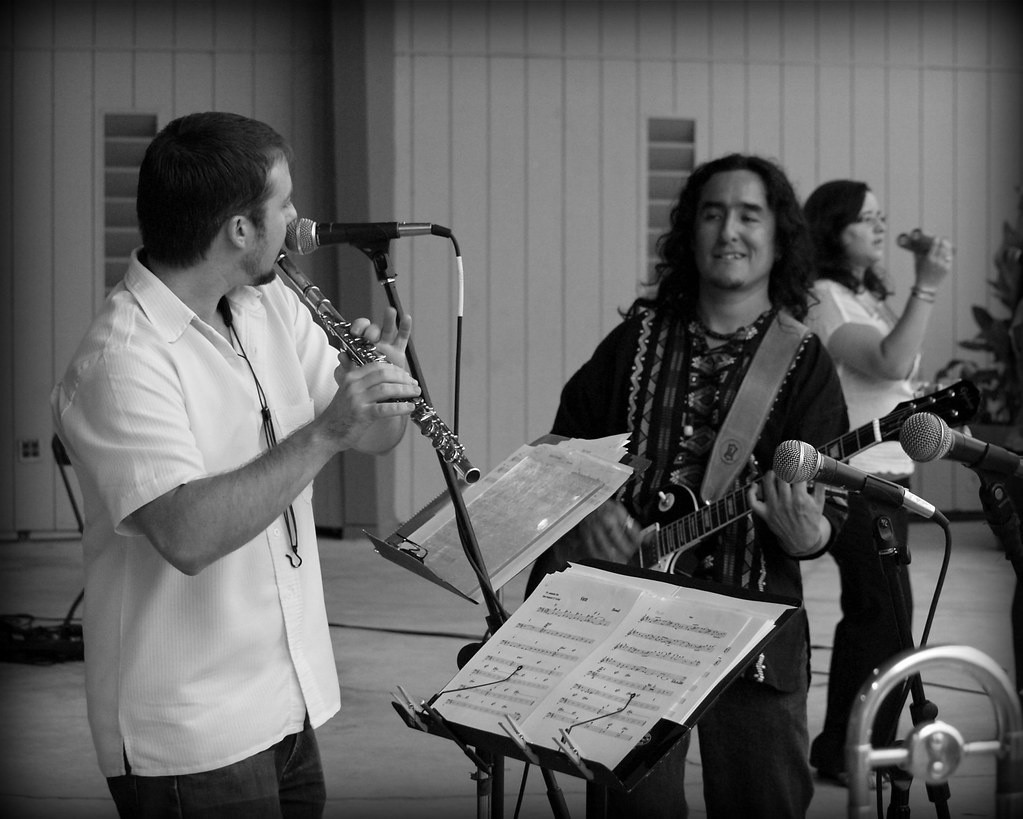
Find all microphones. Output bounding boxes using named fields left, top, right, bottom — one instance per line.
left=284, top=216, right=431, bottom=256
left=900, top=411, right=1023, bottom=480
left=773, top=440, right=936, bottom=520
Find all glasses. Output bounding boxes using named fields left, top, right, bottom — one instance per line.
left=855, top=209, right=884, bottom=220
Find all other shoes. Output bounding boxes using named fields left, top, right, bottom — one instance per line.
left=819, top=766, right=886, bottom=791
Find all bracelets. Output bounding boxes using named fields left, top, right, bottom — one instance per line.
left=912, top=285, right=936, bottom=303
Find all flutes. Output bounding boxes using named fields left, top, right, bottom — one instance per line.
left=273, top=253, right=482, bottom=487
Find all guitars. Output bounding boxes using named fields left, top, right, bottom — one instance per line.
left=521, top=381, right=983, bottom=599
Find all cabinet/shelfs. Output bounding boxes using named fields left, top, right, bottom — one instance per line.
left=98, top=108, right=160, bottom=302
left=641, top=115, right=697, bottom=299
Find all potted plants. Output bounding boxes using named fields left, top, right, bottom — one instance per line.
left=936, top=186, right=1023, bottom=533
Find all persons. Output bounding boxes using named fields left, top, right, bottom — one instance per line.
left=51, top=112, right=421, bottom=819
left=552, top=156, right=849, bottom=818
left=804, top=179, right=955, bottom=788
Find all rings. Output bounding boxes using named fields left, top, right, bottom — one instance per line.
left=946, top=258, right=952, bottom=261
left=624, top=515, right=634, bottom=535
